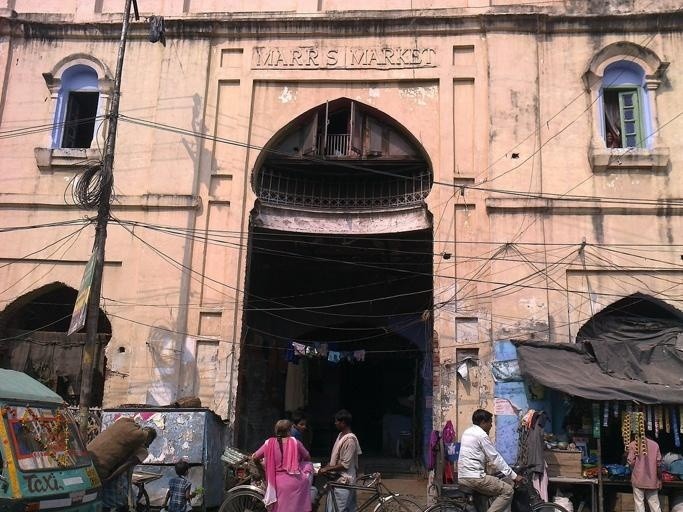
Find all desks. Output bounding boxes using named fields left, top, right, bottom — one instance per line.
left=547, top=475, right=683, bottom=512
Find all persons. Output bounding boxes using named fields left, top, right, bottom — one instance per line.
left=161, top=461, right=198, bottom=512
left=427, top=430, right=442, bottom=477
left=606, top=131, right=614, bottom=148
left=626, top=422, right=664, bottom=512
left=251, top=419, right=314, bottom=512
left=289, top=410, right=309, bottom=442
left=457, top=408, right=527, bottom=512
left=319, top=408, right=363, bottom=512
left=98, top=414, right=158, bottom=512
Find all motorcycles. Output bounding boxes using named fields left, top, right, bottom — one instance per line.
left=423, top=462, right=568, bottom=512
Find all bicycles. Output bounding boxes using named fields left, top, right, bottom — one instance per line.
left=219, top=469, right=421, bottom=512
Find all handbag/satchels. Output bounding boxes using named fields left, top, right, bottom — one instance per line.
left=512, top=477, right=541, bottom=511
